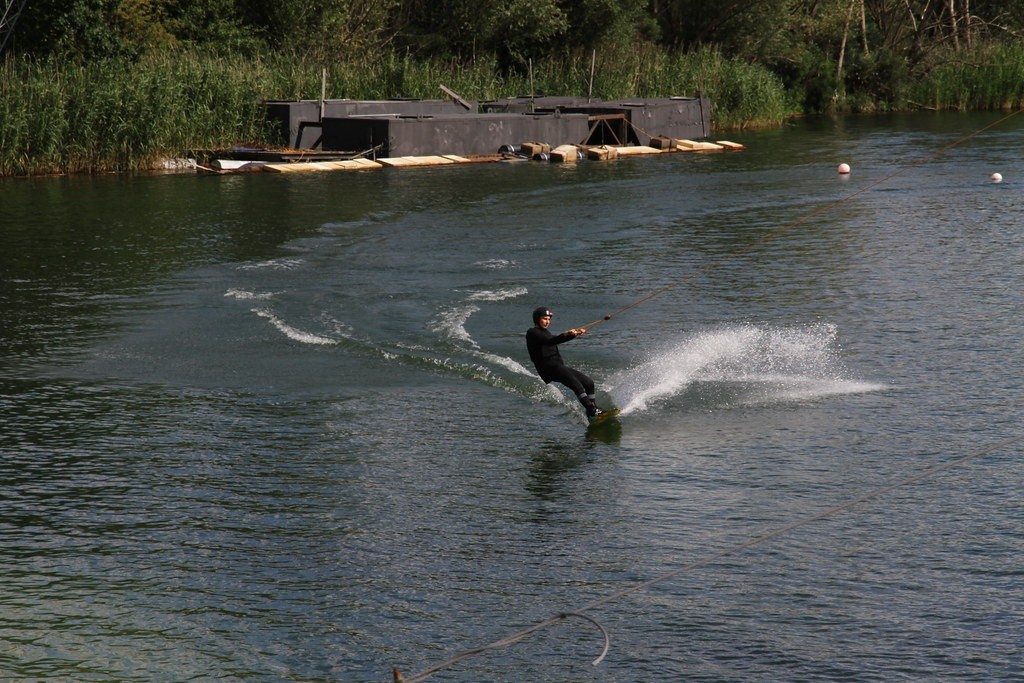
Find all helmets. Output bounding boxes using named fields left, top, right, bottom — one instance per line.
left=533, top=307, right=553, bottom=325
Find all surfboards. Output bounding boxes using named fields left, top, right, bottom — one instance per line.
left=586, top=390, right=620, bottom=426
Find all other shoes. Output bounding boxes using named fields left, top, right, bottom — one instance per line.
left=587, top=409, right=607, bottom=422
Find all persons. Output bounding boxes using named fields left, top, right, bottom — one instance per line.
left=526, top=306, right=606, bottom=420
